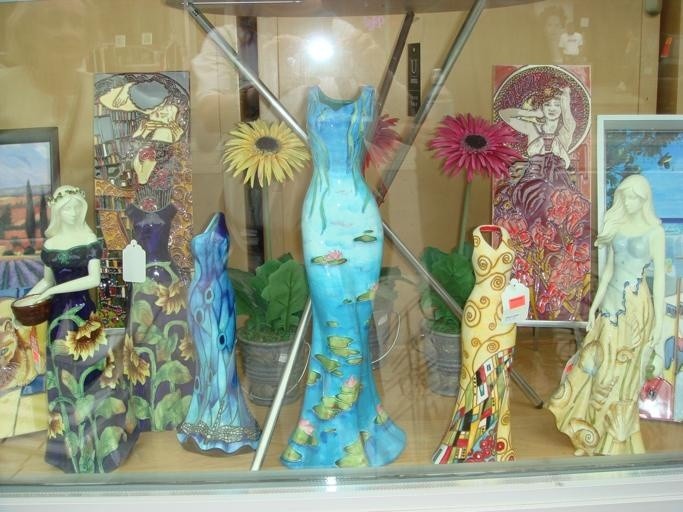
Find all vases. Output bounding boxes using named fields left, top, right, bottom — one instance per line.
left=237, top=324, right=310, bottom=407
left=421, top=326, right=462, bottom=398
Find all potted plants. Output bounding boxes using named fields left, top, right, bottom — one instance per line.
left=368, top=267, right=404, bottom=370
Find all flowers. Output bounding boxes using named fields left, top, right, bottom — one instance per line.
left=224, top=118, right=314, bottom=338
left=128, top=262, right=195, bottom=431
left=417, top=114, right=522, bottom=333
left=44, top=303, right=136, bottom=473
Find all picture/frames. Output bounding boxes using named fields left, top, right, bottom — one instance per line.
left=0, top=128, right=62, bottom=254
left=596, top=115, right=683, bottom=423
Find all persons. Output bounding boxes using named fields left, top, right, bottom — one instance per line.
left=544, top=175, right=666, bottom=456
left=497, top=87, right=590, bottom=247
left=559, top=24, right=584, bottom=64
left=0, top=0, right=111, bottom=198
left=11, top=184, right=141, bottom=475
left=190, top=0, right=408, bottom=272
left=538, top=7, right=566, bottom=66
